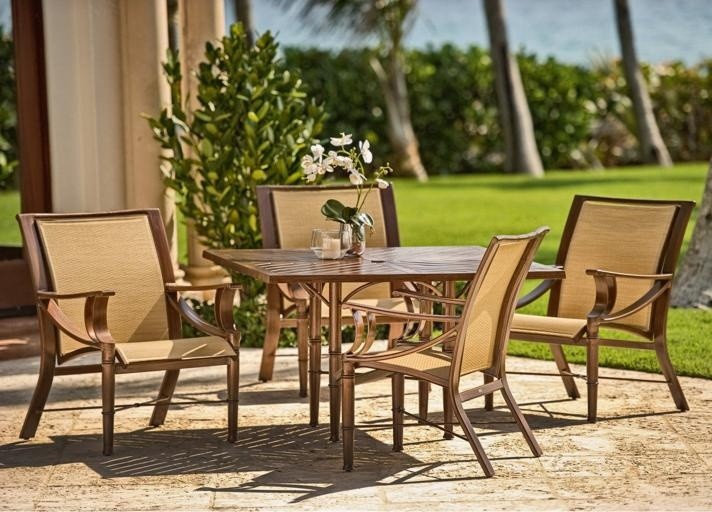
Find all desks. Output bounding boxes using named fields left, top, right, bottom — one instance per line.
left=202, top=245, right=566, bottom=441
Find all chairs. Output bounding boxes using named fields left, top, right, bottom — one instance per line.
left=253, top=183, right=420, bottom=398
left=483, top=194, right=696, bottom=424
left=15, top=208, right=244, bottom=456
left=342, top=226, right=550, bottom=478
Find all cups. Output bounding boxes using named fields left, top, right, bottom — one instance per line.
left=310, top=228, right=350, bottom=261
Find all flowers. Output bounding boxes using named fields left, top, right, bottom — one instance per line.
left=300, top=133, right=394, bottom=243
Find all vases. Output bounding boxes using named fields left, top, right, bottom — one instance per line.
left=340, top=222, right=365, bottom=257
